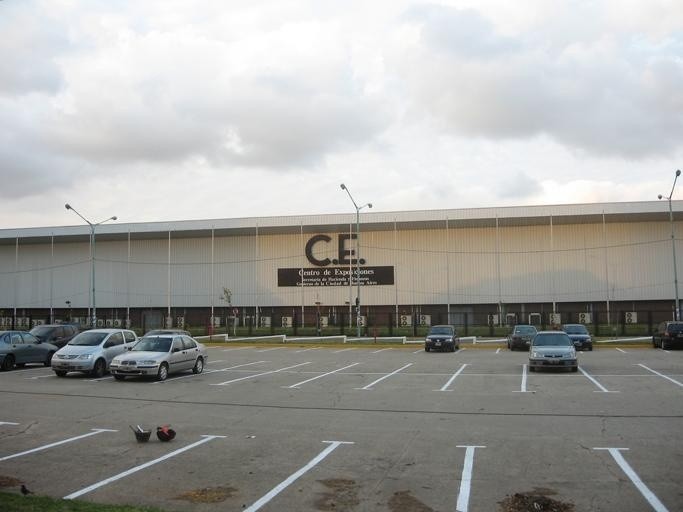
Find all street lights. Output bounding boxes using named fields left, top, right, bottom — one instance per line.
left=657, top=169, right=681, bottom=320
left=340, top=183, right=373, bottom=338
left=65, top=202, right=118, bottom=327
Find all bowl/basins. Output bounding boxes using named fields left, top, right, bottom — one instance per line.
left=135, top=430, right=151, bottom=441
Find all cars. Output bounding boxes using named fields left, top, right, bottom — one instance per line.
left=0, top=325, right=208, bottom=381
left=506, top=324, right=593, bottom=372
left=425, top=325, right=459, bottom=352
left=653, top=320, right=683, bottom=349
